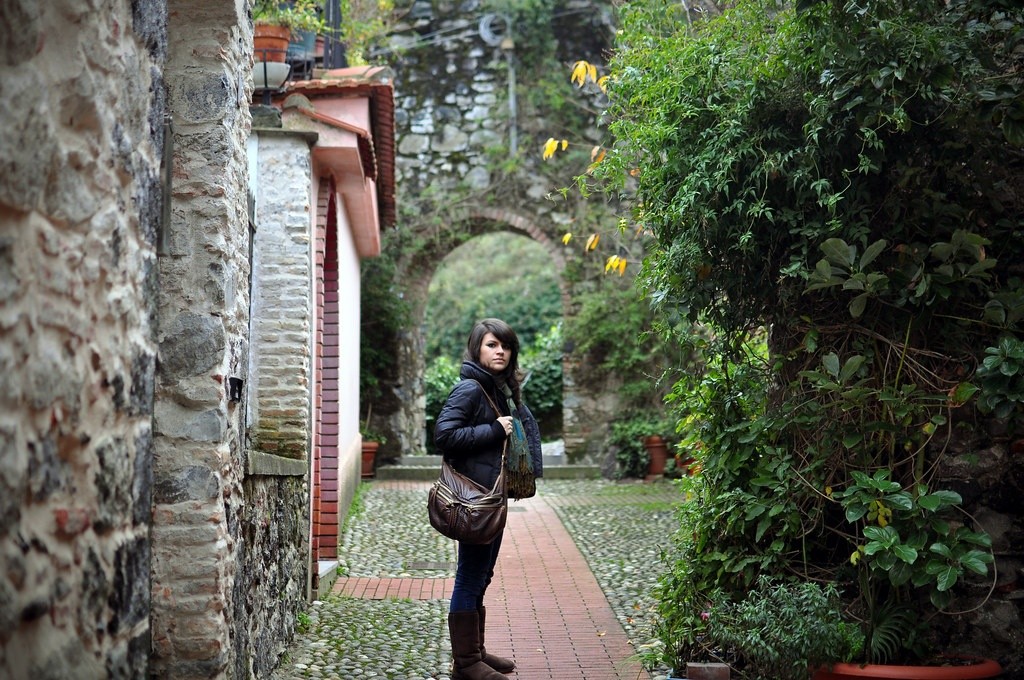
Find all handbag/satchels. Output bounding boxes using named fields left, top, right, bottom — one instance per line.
left=427, top=459, right=509, bottom=544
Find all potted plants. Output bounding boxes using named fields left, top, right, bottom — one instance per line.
left=253, top=0, right=332, bottom=64
left=360, top=420, right=388, bottom=480
left=805, top=467, right=1004, bottom=680
left=600, top=414, right=684, bottom=480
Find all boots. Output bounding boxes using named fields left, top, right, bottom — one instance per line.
left=449, top=610, right=510, bottom=680
left=477, top=606, right=515, bottom=673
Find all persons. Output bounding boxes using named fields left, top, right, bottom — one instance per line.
left=432, top=316, right=545, bottom=680
